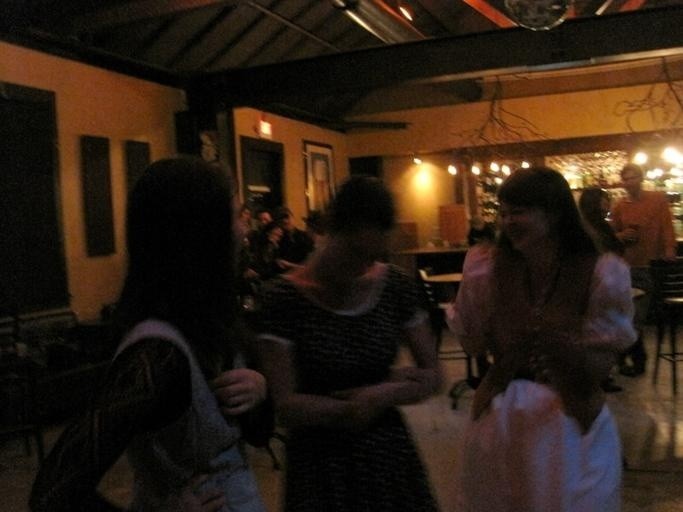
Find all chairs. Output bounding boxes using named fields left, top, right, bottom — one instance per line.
left=0, top=312, right=108, bottom=462
left=648, top=256, right=683, bottom=396
left=417, top=268, right=450, bottom=357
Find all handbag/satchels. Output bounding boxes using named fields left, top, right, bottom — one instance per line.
left=29, top=412, right=264, bottom=512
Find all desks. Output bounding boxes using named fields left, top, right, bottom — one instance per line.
left=427, top=273, right=493, bottom=410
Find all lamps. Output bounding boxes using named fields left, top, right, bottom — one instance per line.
left=613, top=56, right=683, bottom=187
left=448, top=75, right=548, bottom=185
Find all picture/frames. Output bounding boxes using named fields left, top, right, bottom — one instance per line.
left=303, top=139, right=337, bottom=214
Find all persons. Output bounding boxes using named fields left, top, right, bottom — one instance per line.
left=461, top=165, right=668, bottom=398
left=445, top=167, right=634, bottom=510
left=238, top=205, right=318, bottom=447
left=252, top=175, right=449, bottom=510
left=22, top=154, right=282, bottom=509
